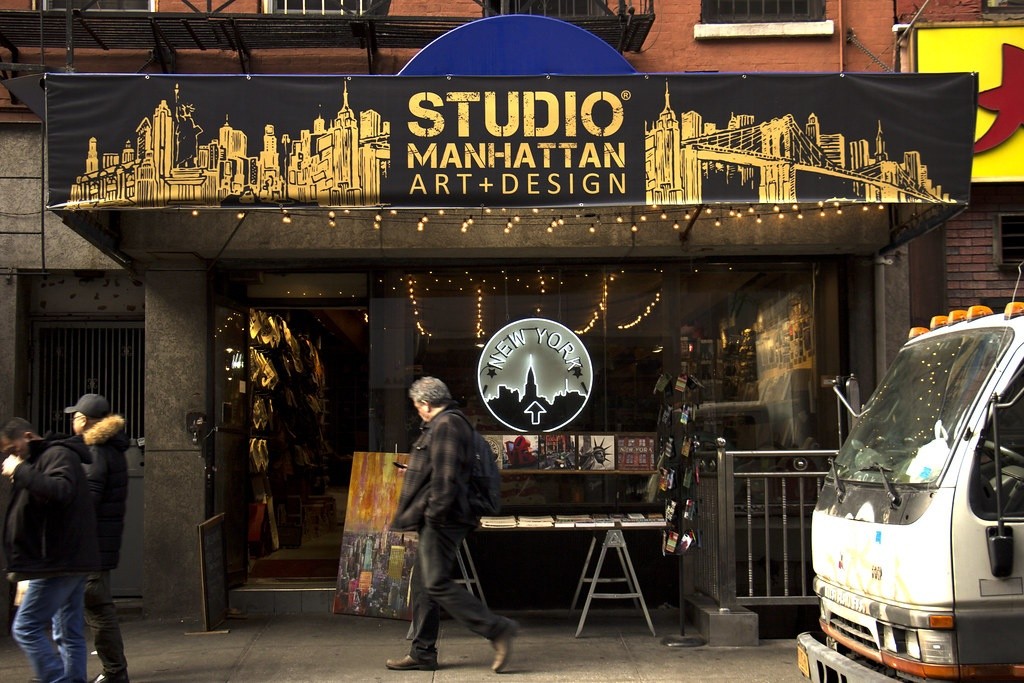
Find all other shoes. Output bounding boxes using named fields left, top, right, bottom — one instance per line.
left=88, top=665, right=129, bottom=683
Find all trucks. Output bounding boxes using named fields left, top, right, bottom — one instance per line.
left=795, top=302, right=1024, bottom=683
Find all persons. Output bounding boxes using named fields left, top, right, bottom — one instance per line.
left=0, top=416, right=94, bottom=683
left=64, top=393, right=132, bottom=683
left=386, top=376, right=518, bottom=672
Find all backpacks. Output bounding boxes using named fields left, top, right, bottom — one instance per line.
left=430, top=407, right=500, bottom=515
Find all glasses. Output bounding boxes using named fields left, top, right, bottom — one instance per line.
left=69, top=414, right=83, bottom=421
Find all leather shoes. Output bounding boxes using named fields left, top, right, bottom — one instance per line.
left=491, top=615, right=519, bottom=672
left=386, top=655, right=438, bottom=671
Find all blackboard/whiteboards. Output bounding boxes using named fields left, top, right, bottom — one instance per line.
left=197, top=512, right=230, bottom=631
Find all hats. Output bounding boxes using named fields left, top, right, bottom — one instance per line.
left=63, top=393, right=109, bottom=418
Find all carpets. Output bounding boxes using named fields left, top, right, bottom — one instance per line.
left=249, top=558, right=339, bottom=577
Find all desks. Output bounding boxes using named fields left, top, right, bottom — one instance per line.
left=406, top=517, right=668, bottom=639
